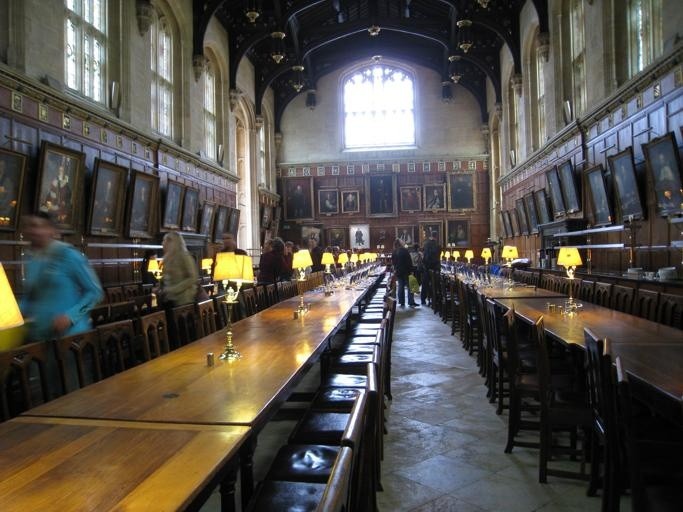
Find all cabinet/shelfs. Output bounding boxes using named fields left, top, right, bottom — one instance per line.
left=553, top=215, right=641, bottom=274
left=537, top=217, right=586, bottom=271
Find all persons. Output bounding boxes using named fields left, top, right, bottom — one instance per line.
left=657, top=152, right=675, bottom=202
left=324, top=194, right=336, bottom=211
left=375, top=176, right=391, bottom=213
left=132, top=186, right=145, bottom=220
left=332, top=233, right=344, bottom=247
left=152, top=230, right=200, bottom=345
left=0, top=159, right=17, bottom=226
left=399, top=228, right=411, bottom=244
left=426, top=225, right=434, bottom=237
left=96, top=178, right=113, bottom=225
left=615, top=162, right=639, bottom=211
left=354, top=228, right=365, bottom=246
left=402, top=191, right=418, bottom=210
left=535, top=248, right=548, bottom=268
left=209, top=232, right=253, bottom=290
left=451, top=224, right=466, bottom=241
left=427, top=188, right=443, bottom=208
left=258, top=236, right=352, bottom=284
left=166, top=191, right=177, bottom=224
left=344, top=193, right=357, bottom=211
left=43, top=162, right=72, bottom=224
left=288, top=184, right=307, bottom=218
left=392, top=231, right=442, bottom=308
left=16, top=207, right=105, bottom=399
left=138, top=248, right=161, bottom=284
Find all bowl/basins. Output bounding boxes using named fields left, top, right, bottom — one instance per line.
left=658, top=267, right=677, bottom=280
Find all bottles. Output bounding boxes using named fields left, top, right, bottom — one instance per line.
left=293, top=311, right=297, bottom=319
left=206, top=353, right=213, bottom=366
left=546, top=302, right=562, bottom=313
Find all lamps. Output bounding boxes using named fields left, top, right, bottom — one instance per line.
left=444, top=245, right=520, bottom=287
left=555, top=245, right=583, bottom=313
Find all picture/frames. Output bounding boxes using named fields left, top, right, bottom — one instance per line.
left=498, top=128, right=682, bottom=244
left=0, top=134, right=242, bottom=244
left=258, top=171, right=478, bottom=253
